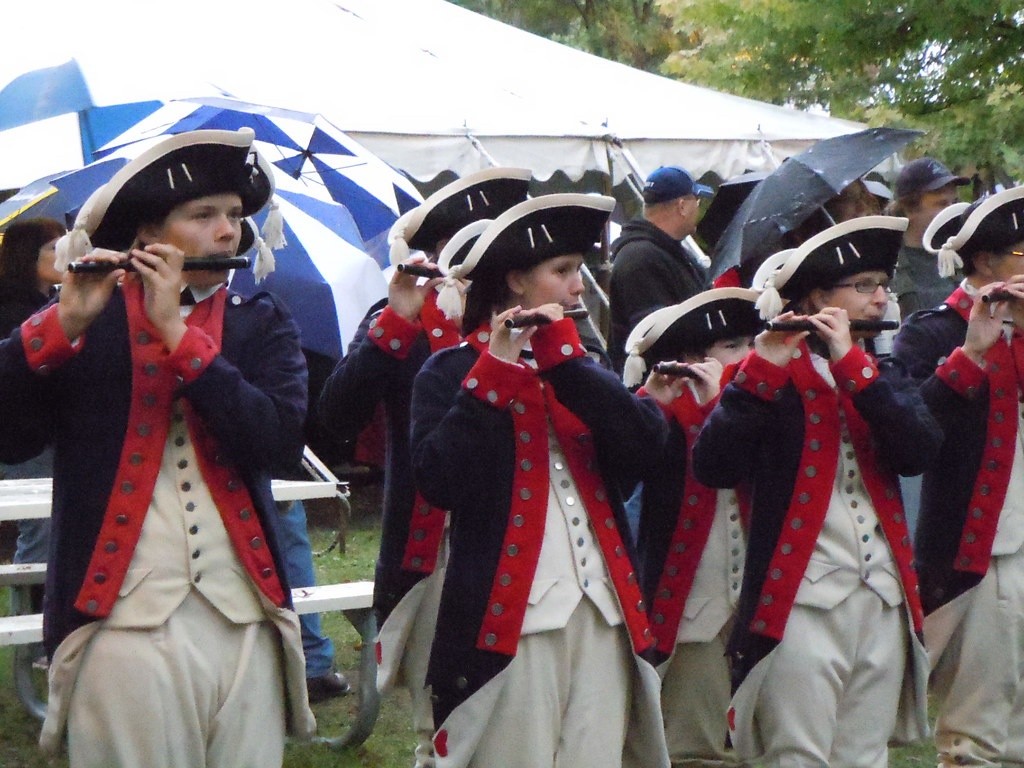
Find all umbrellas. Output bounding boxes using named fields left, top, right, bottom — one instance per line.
left=705, top=128, right=925, bottom=286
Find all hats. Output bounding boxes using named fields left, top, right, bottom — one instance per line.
left=922, top=186, right=1024, bottom=278
left=753, top=216, right=910, bottom=319
left=896, top=157, right=970, bottom=198
left=387, top=168, right=533, bottom=268
left=642, top=165, right=715, bottom=203
left=52, top=127, right=288, bottom=285
left=622, top=286, right=791, bottom=389
left=435, top=193, right=616, bottom=320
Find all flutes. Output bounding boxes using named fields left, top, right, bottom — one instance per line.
left=66, top=256, right=252, bottom=272
left=982, top=292, right=1013, bottom=302
left=653, top=363, right=702, bottom=380
left=505, top=310, right=589, bottom=328
left=397, top=262, right=443, bottom=279
left=765, top=319, right=899, bottom=332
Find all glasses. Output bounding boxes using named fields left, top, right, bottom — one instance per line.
left=821, top=276, right=897, bottom=294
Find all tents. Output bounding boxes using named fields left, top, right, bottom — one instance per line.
left=0, top=0, right=897, bottom=363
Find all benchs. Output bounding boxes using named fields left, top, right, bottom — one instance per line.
left=0, top=559, right=379, bottom=752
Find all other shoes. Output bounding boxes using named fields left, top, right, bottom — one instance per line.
left=306, top=670, right=350, bottom=703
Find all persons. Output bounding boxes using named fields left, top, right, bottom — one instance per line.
left=314, top=167, right=533, bottom=768
left=892, top=183, right=1024, bottom=768
left=0, top=157, right=973, bottom=696
left=688, top=215, right=943, bottom=768
left=408, top=191, right=673, bottom=768
left=0, top=127, right=360, bottom=768
left=620, top=286, right=748, bottom=768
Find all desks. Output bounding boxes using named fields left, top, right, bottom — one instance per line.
left=0, top=478, right=352, bottom=521
